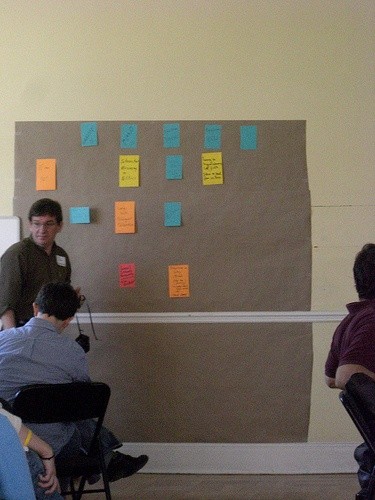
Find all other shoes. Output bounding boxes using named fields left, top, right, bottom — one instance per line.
left=106, top=451, right=149, bottom=482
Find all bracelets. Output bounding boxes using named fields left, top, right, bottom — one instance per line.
left=23, top=429, right=32, bottom=446
left=39, top=453, right=54, bottom=461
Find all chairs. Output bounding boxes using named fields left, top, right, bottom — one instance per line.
left=339, top=372, right=375, bottom=500
left=0, top=382, right=111, bottom=500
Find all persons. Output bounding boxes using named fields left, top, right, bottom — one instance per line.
left=323, top=242, right=375, bottom=389
left=0, top=197, right=84, bottom=332
left=0, top=404, right=66, bottom=500
left=0, top=279, right=151, bottom=489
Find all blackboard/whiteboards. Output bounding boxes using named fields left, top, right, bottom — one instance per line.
left=0, top=215, right=20, bottom=256
